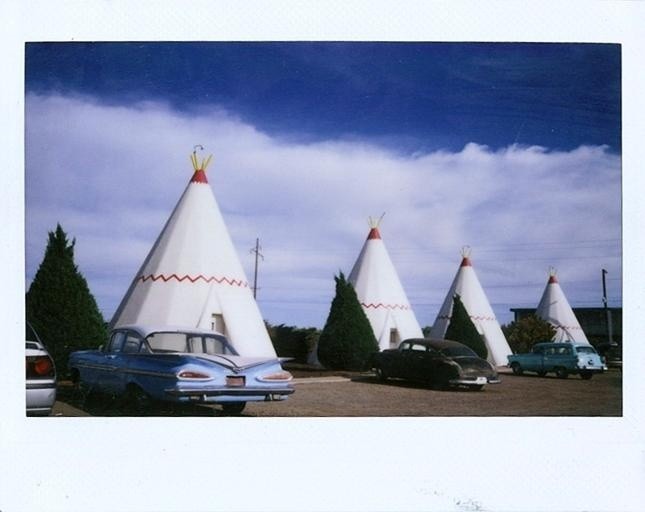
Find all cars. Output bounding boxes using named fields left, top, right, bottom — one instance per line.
left=507, top=341, right=608, bottom=378
left=369, top=339, right=502, bottom=391
left=68, top=324, right=296, bottom=416
left=25, top=318, right=55, bottom=414
